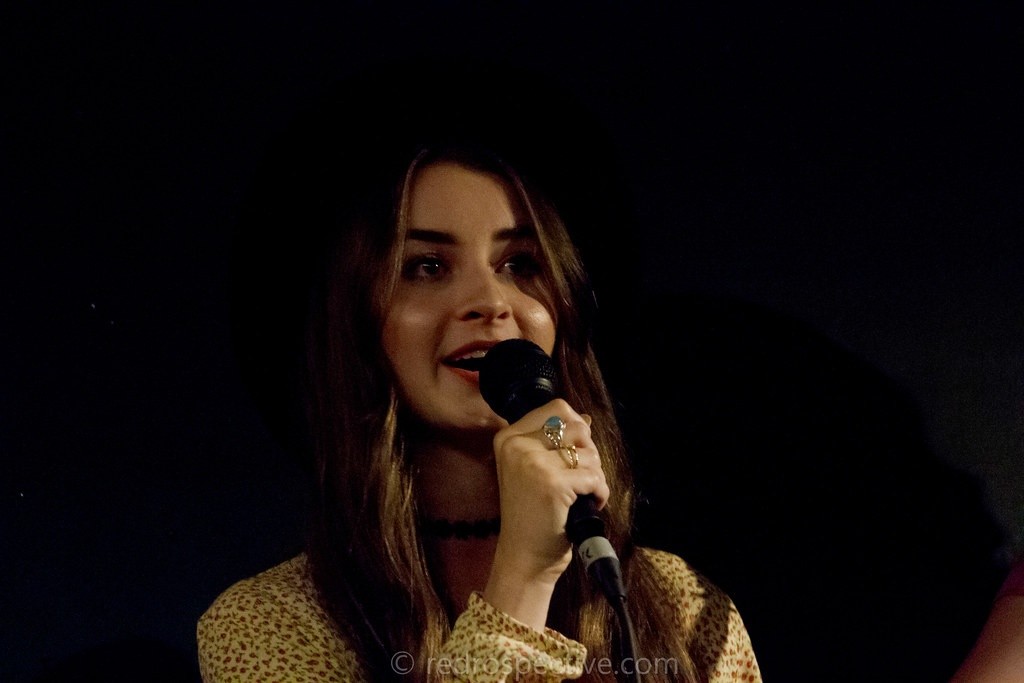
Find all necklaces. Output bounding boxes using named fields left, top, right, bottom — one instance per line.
left=422, top=514, right=501, bottom=538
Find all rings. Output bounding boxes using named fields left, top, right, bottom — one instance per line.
left=541, top=416, right=566, bottom=450
left=563, top=443, right=579, bottom=469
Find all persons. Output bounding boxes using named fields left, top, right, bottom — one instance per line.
left=194, top=119, right=763, bottom=683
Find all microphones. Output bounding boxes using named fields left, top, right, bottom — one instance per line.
left=477, top=338, right=626, bottom=605
